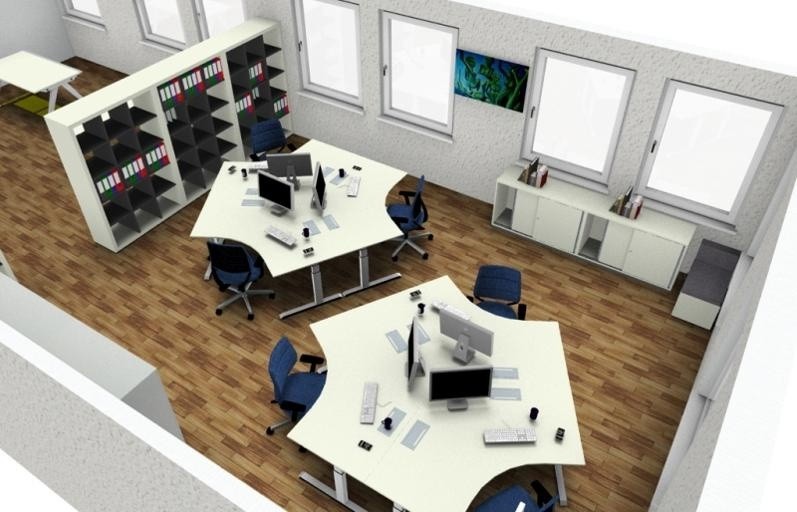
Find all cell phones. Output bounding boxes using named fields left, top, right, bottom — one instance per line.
left=228, top=166, right=236, bottom=171
left=555, top=427, right=567, bottom=441
left=353, top=165, right=361, bottom=171
left=357, top=440, right=373, bottom=453
left=303, top=247, right=313, bottom=254
left=409, top=289, right=422, bottom=297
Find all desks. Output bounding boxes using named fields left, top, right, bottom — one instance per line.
left=1, top=49, right=86, bottom=115
left=189, top=138, right=407, bottom=320
left=287, top=273, right=588, bottom=512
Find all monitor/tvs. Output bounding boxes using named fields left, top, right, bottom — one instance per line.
left=265, top=151, right=313, bottom=191
left=256, top=169, right=296, bottom=215
left=438, top=302, right=494, bottom=364
left=427, top=366, right=493, bottom=411
left=310, top=161, right=326, bottom=212
left=405, top=315, right=426, bottom=385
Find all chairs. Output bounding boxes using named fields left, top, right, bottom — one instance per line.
left=465, top=264, right=526, bottom=318
left=266, top=337, right=328, bottom=434
left=205, top=240, right=275, bottom=321
left=387, top=175, right=434, bottom=261
left=472, top=480, right=561, bottom=512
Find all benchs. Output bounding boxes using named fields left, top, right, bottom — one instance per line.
left=669, top=238, right=742, bottom=330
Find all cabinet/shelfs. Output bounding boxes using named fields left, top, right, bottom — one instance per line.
left=490, top=166, right=702, bottom=292
left=43, top=18, right=296, bottom=252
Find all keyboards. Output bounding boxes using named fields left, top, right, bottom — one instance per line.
left=249, top=168, right=270, bottom=173
left=482, top=427, right=537, bottom=444
left=431, top=299, right=470, bottom=321
left=345, top=176, right=361, bottom=197
left=360, top=382, right=378, bottom=424
left=265, top=224, right=297, bottom=247
